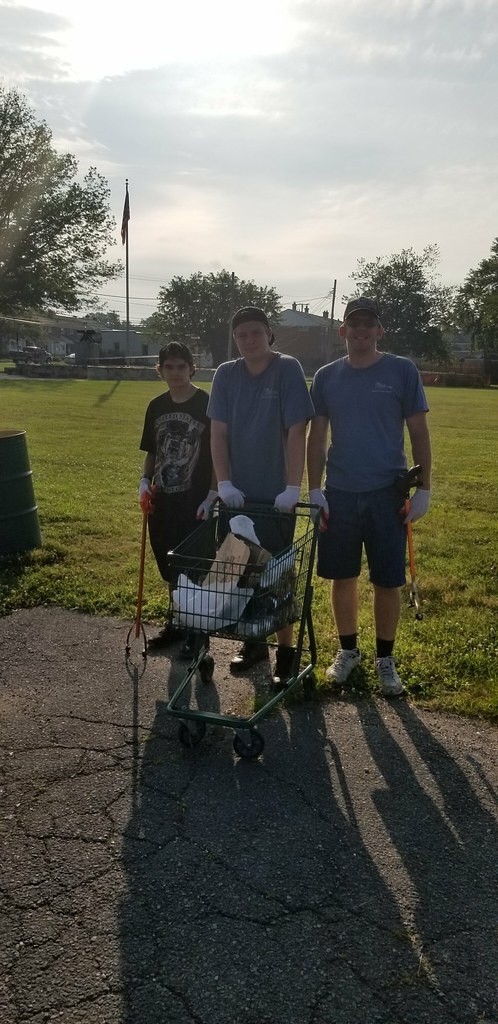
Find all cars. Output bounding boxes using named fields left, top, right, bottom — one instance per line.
left=65, top=352, right=75, bottom=364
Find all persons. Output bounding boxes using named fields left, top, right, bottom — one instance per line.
left=139, top=342, right=219, bottom=661
left=307, top=298, right=433, bottom=695
left=206, top=307, right=316, bottom=691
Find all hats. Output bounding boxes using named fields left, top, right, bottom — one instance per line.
left=231, top=307, right=274, bottom=346
left=344, top=297, right=380, bottom=321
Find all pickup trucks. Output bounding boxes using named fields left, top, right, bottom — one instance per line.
left=11, top=345, right=53, bottom=364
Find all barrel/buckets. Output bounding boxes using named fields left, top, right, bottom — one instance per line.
left=0, top=429, right=41, bottom=554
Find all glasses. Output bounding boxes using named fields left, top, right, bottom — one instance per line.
left=346, top=320, right=379, bottom=329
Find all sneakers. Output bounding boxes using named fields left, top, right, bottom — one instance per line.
left=231, top=638, right=270, bottom=672
left=326, top=646, right=361, bottom=683
left=375, top=651, right=403, bottom=696
left=175, top=632, right=210, bottom=659
left=272, top=648, right=297, bottom=685
left=147, top=625, right=183, bottom=648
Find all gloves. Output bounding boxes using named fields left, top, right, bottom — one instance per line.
left=217, top=480, right=246, bottom=510
left=309, top=489, right=329, bottom=534
left=139, top=478, right=156, bottom=517
left=196, top=489, right=218, bottom=521
left=273, top=485, right=300, bottom=515
left=401, top=488, right=429, bottom=525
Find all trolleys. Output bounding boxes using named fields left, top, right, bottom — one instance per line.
left=167, top=495, right=325, bottom=760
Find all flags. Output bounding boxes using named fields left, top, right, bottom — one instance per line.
left=121, top=191, right=130, bottom=246
